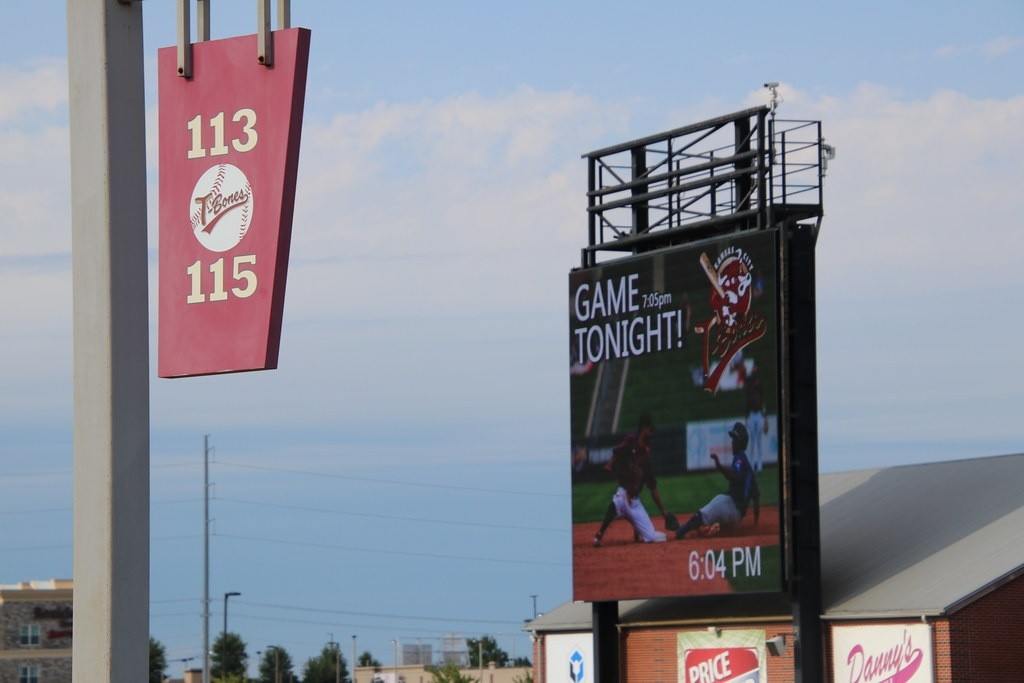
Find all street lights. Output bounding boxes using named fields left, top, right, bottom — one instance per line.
left=530, top=594, right=536, bottom=620
left=221, top=591, right=242, bottom=674
left=266, top=645, right=279, bottom=683
left=472, top=640, right=483, bottom=683
left=351, top=635, right=357, bottom=683
left=390, top=639, right=398, bottom=683
left=327, top=641, right=340, bottom=683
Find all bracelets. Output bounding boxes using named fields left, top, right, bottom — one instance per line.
left=763, top=412, right=767, bottom=418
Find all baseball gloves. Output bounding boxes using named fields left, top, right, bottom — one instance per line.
left=664, top=511, right=680, bottom=531
left=763, top=419, right=769, bottom=434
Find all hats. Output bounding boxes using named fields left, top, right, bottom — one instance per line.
left=728, top=422, right=748, bottom=448
left=637, top=411, right=658, bottom=427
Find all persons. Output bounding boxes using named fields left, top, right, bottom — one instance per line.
left=745, top=376, right=769, bottom=476
left=593, top=411, right=678, bottom=546
left=676, top=423, right=761, bottom=539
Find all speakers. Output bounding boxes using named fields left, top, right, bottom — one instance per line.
left=766, top=637, right=786, bottom=657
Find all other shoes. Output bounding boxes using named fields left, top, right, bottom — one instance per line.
left=593, top=532, right=603, bottom=545
left=702, top=523, right=720, bottom=537
left=634, top=528, right=640, bottom=543
left=676, top=527, right=683, bottom=538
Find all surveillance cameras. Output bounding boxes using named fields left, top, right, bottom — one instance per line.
left=764, top=82, right=779, bottom=88
